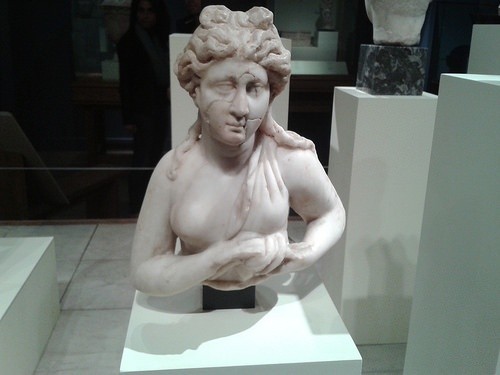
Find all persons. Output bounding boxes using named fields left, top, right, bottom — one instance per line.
left=129, top=5, right=346, bottom=298
